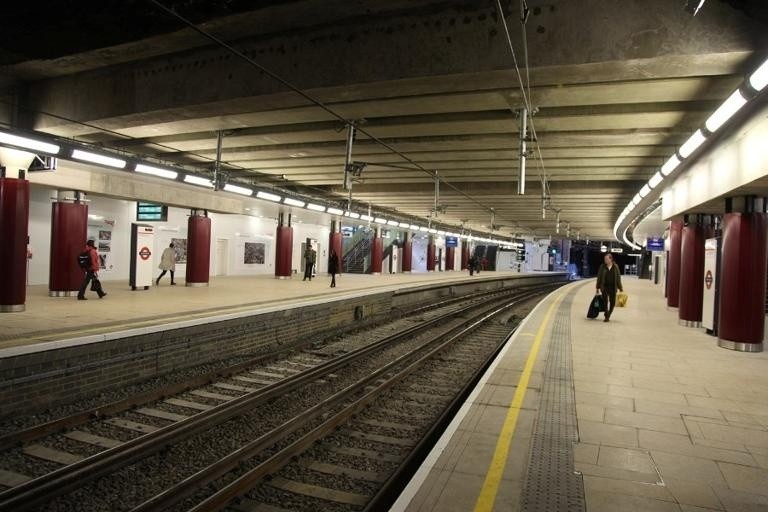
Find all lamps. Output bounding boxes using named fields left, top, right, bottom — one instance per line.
left=683, top=0, right=705, bottom=17
left=612, top=57, right=768, bottom=250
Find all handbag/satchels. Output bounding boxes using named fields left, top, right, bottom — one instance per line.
left=585, top=294, right=607, bottom=318
left=614, top=293, right=629, bottom=307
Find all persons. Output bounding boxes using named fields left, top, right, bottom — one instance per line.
left=596, top=253, right=623, bottom=322
left=302, top=244, right=316, bottom=281
left=328, top=248, right=338, bottom=287
left=156, top=243, right=176, bottom=285
left=470, top=256, right=488, bottom=275
left=77, top=239, right=107, bottom=300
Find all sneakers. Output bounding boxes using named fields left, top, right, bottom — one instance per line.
left=602, top=313, right=612, bottom=322
